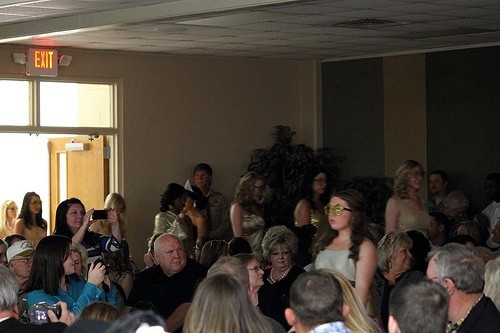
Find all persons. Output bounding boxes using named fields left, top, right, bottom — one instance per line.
left=0, top=161, right=500, bottom=333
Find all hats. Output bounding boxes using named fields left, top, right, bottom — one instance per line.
left=6, top=240, right=37, bottom=263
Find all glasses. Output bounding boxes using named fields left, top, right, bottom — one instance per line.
left=11, top=258, right=34, bottom=264
left=253, top=185, right=266, bottom=191
left=324, top=204, right=352, bottom=216
left=314, top=178, right=329, bottom=184
left=248, top=266, right=264, bottom=271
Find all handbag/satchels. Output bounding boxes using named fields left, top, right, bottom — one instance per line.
left=112, top=271, right=134, bottom=300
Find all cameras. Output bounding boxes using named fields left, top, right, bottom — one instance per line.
left=28, top=301, right=62, bottom=324
left=94, top=258, right=110, bottom=275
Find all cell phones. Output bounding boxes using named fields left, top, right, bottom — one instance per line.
left=92, top=210, right=108, bottom=219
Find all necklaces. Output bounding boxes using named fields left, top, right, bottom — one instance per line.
left=271, top=266, right=293, bottom=282
